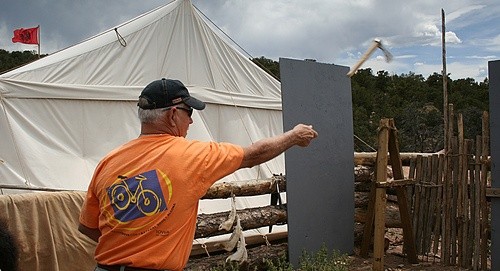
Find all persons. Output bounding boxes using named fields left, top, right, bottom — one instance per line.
left=77, top=78, right=319, bottom=271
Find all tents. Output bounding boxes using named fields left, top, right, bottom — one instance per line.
left=0, top=0, right=287, bottom=246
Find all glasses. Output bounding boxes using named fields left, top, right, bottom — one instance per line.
left=163, top=107, right=193, bottom=116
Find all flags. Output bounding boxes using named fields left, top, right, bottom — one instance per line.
left=12, top=24, right=40, bottom=45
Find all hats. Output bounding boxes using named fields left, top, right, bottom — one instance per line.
left=137, top=77, right=206, bottom=110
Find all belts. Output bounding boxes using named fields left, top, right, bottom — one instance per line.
left=97, top=264, right=165, bottom=271
